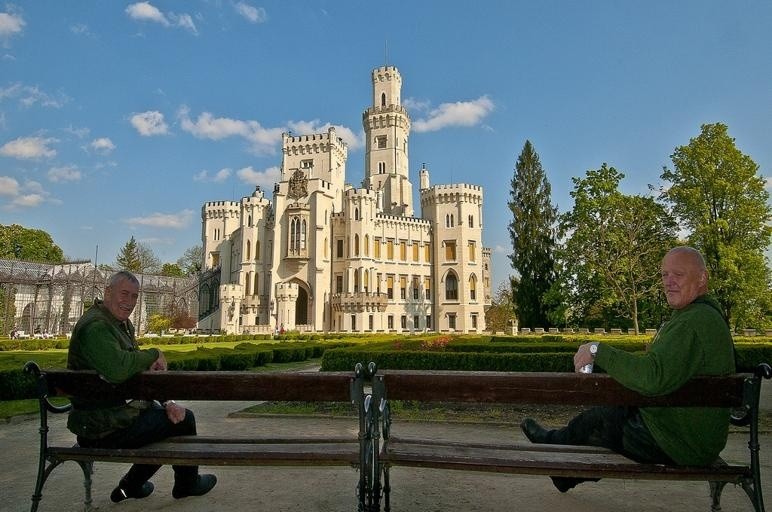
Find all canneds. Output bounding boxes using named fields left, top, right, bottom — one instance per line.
left=578, top=362, right=594, bottom=375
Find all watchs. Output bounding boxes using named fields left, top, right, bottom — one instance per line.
left=589, top=341, right=600, bottom=358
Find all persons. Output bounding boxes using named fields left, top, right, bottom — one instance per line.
left=66, top=268, right=218, bottom=503
left=516, top=245, right=738, bottom=494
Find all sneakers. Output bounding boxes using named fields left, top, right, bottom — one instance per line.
left=172, top=474, right=216, bottom=499
left=521, top=419, right=547, bottom=443
left=111, top=481, right=155, bottom=503
left=549, top=475, right=574, bottom=493
